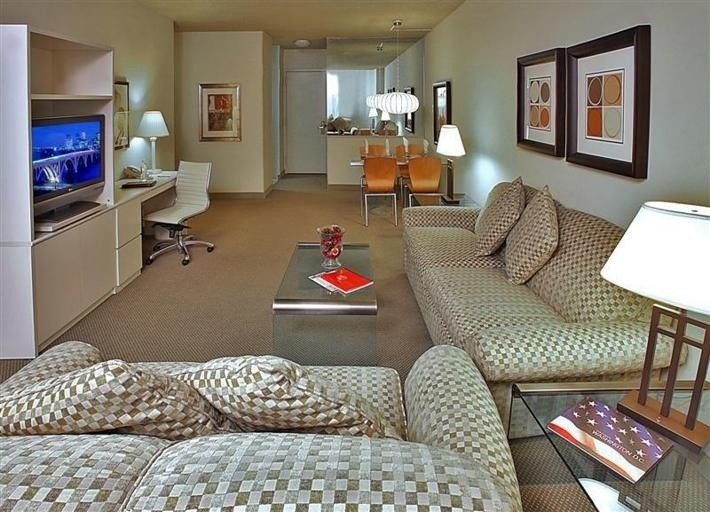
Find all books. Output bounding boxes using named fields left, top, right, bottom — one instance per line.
left=546, top=396, right=674, bottom=485
left=320, top=268, right=374, bottom=294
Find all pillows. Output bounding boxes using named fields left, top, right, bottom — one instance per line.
left=475, top=177, right=526, bottom=256
left=166, top=357, right=399, bottom=438
left=0, top=361, right=240, bottom=437
left=505, top=185, right=559, bottom=286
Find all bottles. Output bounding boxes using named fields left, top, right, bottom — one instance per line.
left=140, top=161, right=145, bottom=180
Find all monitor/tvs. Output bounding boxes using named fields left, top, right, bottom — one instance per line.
left=31, top=113, right=108, bottom=233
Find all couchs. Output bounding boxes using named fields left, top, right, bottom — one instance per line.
left=0, top=340, right=524, bottom=512
left=402, top=182, right=689, bottom=416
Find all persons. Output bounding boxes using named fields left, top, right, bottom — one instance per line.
left=64, top=128, right=102, bottom=149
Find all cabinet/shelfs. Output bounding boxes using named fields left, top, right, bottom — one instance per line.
left=0, top=23, right=116, bottom=359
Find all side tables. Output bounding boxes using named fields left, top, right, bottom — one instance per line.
left=506, top=381, right=709, bottom=512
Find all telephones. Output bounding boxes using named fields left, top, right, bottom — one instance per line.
left=123, top=165, right=145, bottom=179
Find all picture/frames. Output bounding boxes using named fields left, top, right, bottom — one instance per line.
left=516, top=48, right=565, bottom=156
left=198, top=83, right=241, bottom=142
left=114, top=81, right=129, bottom=150
left=432, top=80, right=452, bottom=145
left=387, top=87, right=415, bottom=132
left=564, top=24, right=650, bottom=179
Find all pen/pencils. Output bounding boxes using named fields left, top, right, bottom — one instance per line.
left=157, top=175, right=170, bottom=177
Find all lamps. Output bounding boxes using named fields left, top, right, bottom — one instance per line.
left=366, top=38, right=420, bottom=121
left=134, top=110, right=170, bottom=174
left=436, top=125, right=466, bottom=204
left=381, top=18, right=413, bottom=115
left=600, top=201, right=710, bottom=455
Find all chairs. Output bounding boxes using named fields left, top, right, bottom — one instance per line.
left=143, top=160, right=215, bottom=266
left=360, top=144, right=442, bottom=227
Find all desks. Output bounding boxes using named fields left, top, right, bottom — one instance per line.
left=114, top=170, right=188, bottom=293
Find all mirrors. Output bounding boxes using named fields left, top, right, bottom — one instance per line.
left=327, top=36, right=425, bottom=138
